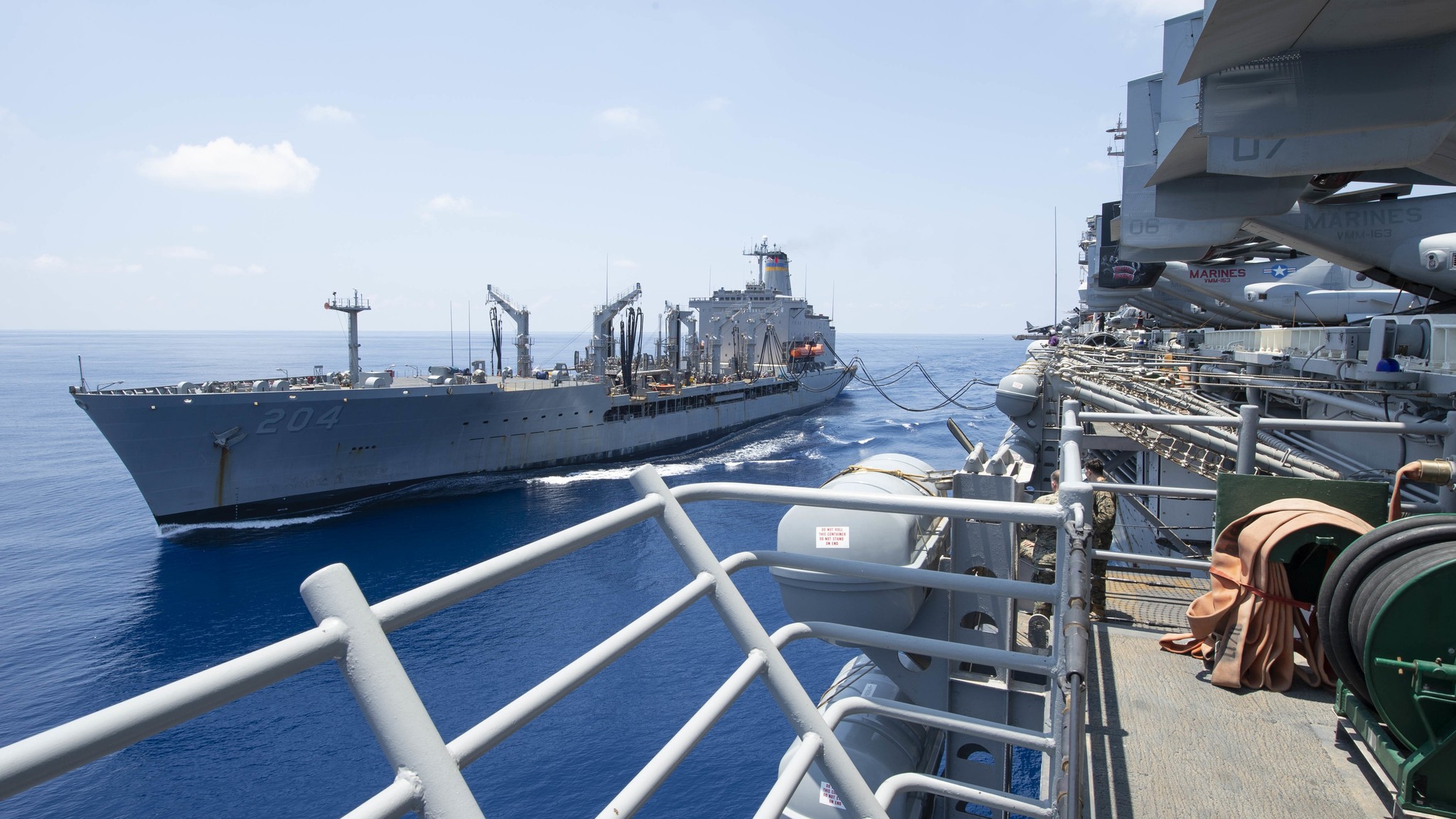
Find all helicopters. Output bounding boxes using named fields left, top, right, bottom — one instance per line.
left=1105, top=307, right=1183, bottom=332
left=1078, top=1, right=1456, bottom=325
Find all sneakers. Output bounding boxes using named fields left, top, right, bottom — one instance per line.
left=1089, top=603, right=1093, bottom=613
left=1089, top=605, right=1106, bottom=622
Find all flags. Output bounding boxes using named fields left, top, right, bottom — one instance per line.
left=775, top=257, right=779, bottom=264
left=767, top=256, right=773, bottom=261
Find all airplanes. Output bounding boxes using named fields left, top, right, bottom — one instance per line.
left=1025, top=320, right=1072, bottom=335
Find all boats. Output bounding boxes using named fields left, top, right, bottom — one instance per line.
left=648, top=383, right=676, bottom=390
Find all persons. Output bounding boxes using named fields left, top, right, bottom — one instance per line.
left=1019, top=469, right=1063, bottom=623
left=1084, top=458, right=1118, bottom=623
left=1134, top=310, right=1144, bottom=330
left=679, top=368, right=775, bottom=386
left=1046, top=328, right=1059, bottom=346
left=1138, top=339, right=1144, bottom=351
left=1097, top=312, right=1105, bottom=332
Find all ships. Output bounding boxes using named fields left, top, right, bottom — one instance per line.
left=68, top=234, right=860, bottom=539
